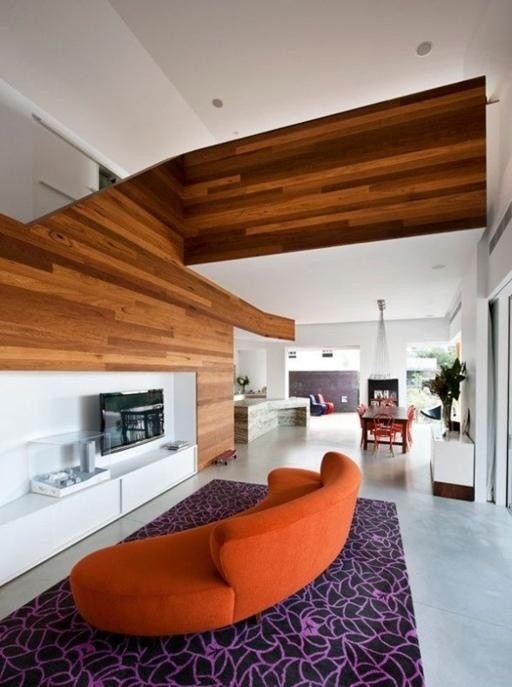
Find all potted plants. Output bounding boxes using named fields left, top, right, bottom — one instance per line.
left=421, top=356, right=467, bottom=436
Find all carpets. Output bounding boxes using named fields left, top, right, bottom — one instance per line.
left=1, top=477, right=429, bottom=686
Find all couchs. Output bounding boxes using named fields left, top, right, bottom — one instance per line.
left=70, top=451, right=363, bottom=637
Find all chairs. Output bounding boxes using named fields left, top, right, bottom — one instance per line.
left=318, top=392, right=334, bottom=414
left=309, top=393, right=326, bottom=416
left=355, top=397, right=417, bottom=457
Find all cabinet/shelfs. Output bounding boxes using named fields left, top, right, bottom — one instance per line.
left=0, top=439, right=199, bottom=590
left=430, top=425, right=474, bottom=500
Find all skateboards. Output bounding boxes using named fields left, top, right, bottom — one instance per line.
left=213, top=450, right=238, bottom=465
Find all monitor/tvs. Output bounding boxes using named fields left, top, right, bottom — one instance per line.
left=99, top=388, right=165, bottom=456
